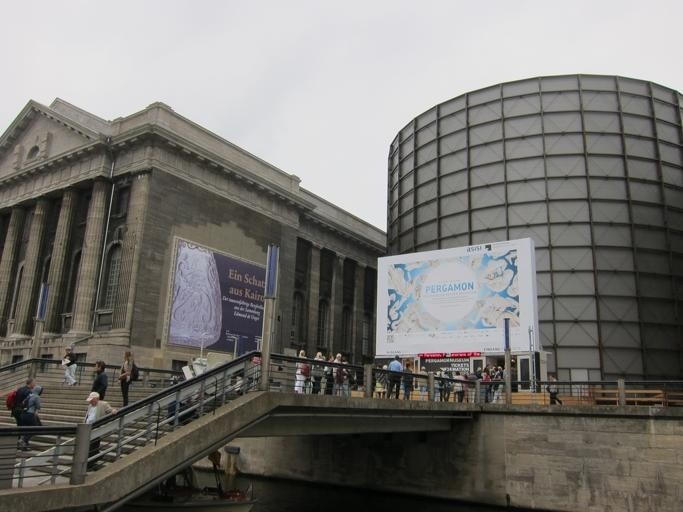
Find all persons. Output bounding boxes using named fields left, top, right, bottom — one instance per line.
left=6, top=377, right=35, bottom=441
left=117, top=350, right=134, bottom=407
left=82, top=392, right=117, bottom=470
left=15, top=384, right=43, bottom=452
left=63, top=347, right=78, bottom=387
left=290, top=346, right=517, bottom=405
left=544, top=374, right=562, bottom=405
left=90, top=359, right=109, bottom=402
left=167, top=376, right=198, bottom=426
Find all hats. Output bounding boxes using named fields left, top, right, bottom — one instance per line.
left=86, top=392, right=99, bottom=401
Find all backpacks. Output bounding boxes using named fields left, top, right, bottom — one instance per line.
left=130, top=364, right=138, bottom=381
left=20, top=396, right=35, bottom=412
left=6, top=390, right=18, bottom=408
left=337, top=368, right=345, bottom=384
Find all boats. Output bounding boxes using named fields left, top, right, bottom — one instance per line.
left=113, top=479, right=260, bottom=512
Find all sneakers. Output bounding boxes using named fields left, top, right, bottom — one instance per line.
left=17, top=439, right=30, bottom=450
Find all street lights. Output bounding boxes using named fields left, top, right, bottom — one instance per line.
left=230, top=335, right=237, bottom=360
left=199, top=331, right=208, bottom=368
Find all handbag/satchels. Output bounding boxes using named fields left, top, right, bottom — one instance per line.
left=301, top=364, right=309, bottom=377
